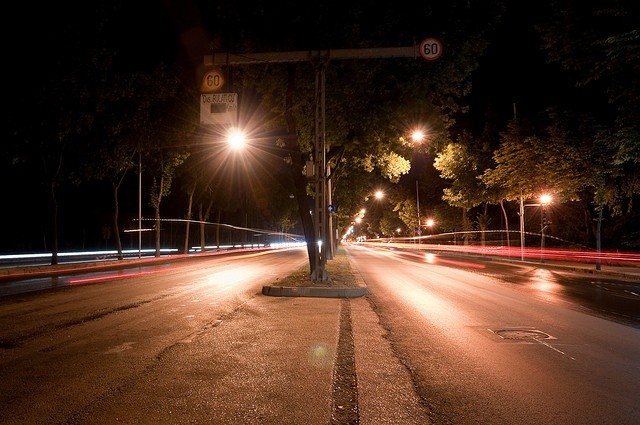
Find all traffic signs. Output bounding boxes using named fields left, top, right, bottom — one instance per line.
left=202, top=70, right=225, bottom=92
left=419, top=37, right=443, bottom=61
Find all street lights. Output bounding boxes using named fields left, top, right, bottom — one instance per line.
left=418, top=218, right=435, bottom=245
left=519, top=193, right=553, bottom=262
